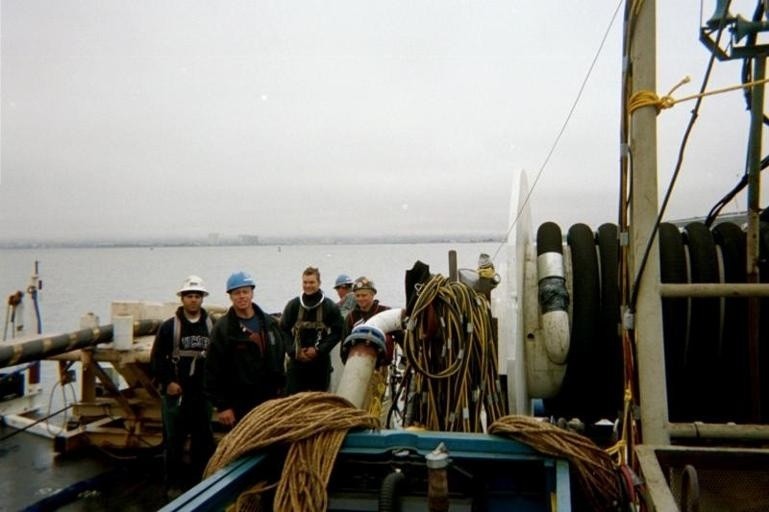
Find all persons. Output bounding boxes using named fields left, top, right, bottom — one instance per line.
left=340, top=277, right=407, bottom=367
left=151, top=274, right=216, bottom=497
left=207, top=273, right=281, bottom=426
left=334, top=274, right=353, bottom=306
left=280, top=266, right=343, bottom=390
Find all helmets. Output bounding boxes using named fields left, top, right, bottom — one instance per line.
left=225, top=270, right=256, bottom=294
left=176, top=276, right=210, bottom=297
left=333, top=274, right=378, bottom=295
